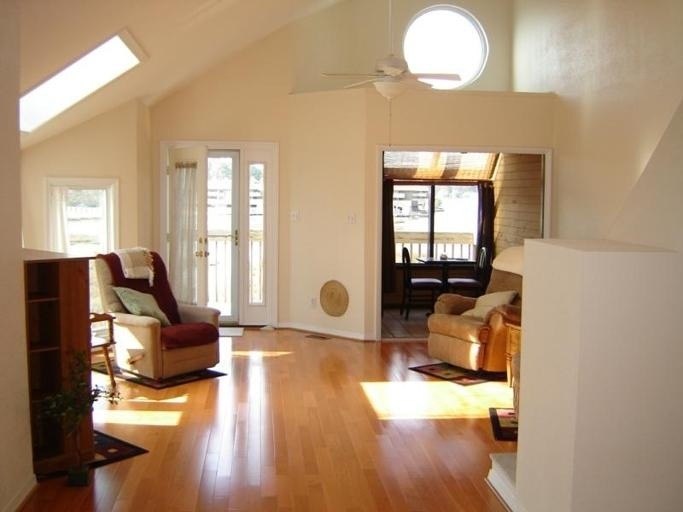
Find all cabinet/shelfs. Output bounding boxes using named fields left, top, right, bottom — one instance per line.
left=23, top=247, right=103, bottom=460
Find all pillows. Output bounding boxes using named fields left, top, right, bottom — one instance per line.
left=461, top=290, right=518, bottom=322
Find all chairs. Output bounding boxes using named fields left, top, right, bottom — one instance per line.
left=400, top=247, right=489, bottom=320
left=94, top=246, right=222, bottom=381
left=427, top=269, right=521, bottom=373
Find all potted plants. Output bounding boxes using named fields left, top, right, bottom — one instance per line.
left=31, top=347, right=123, bottom=488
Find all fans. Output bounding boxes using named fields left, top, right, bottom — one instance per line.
left=318, top=0, right=461, bottom=103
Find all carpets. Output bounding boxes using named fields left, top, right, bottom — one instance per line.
left=489, top=408, right=518, bottom=442
left=37, top=430, right=149, bottom=484
left=92, top=358, right=227, bottom=389
left=407, top=362, right=491, bottom=386
